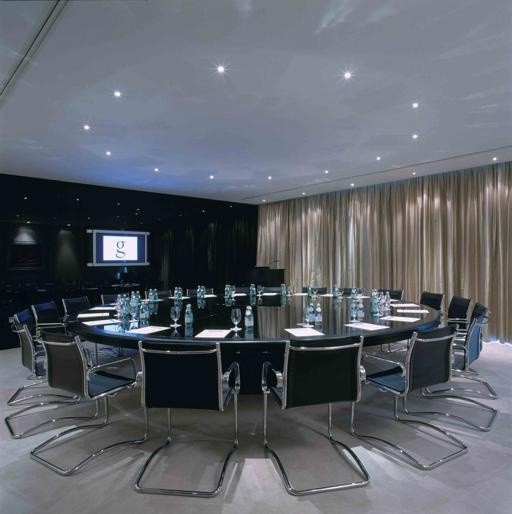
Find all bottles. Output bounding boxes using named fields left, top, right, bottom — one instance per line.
left=110, top=282, right=392, bottom=338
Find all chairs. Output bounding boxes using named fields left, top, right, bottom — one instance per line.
left=4, top=269, right=499, bottom=498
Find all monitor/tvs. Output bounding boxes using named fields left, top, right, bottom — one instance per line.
left=93, top=229, right=148, bottom=266
